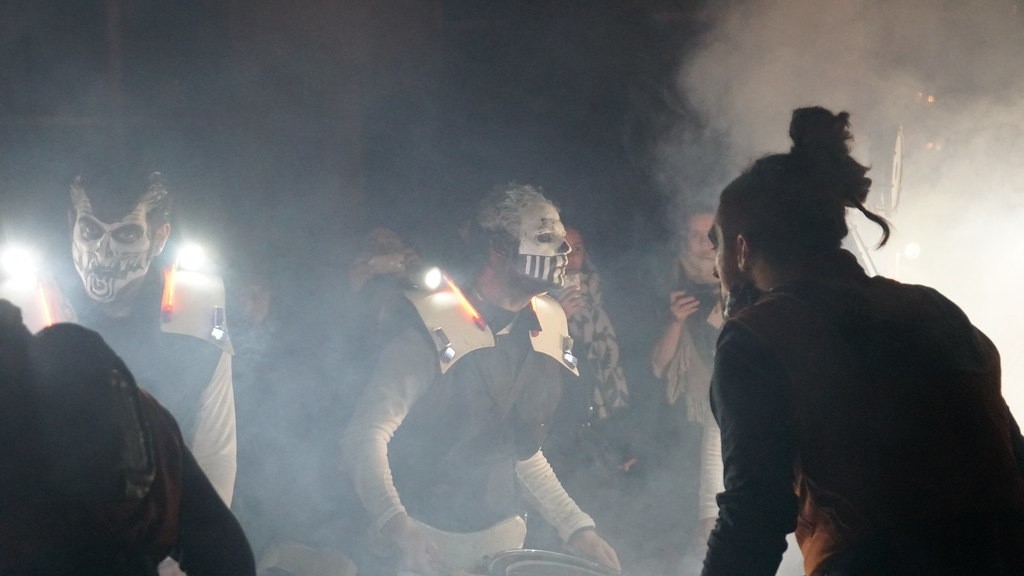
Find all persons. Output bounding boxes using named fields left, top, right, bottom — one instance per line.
left=700, top=104, right=1024, bottom=576
left=0, top=138, right=805, bottom=576
